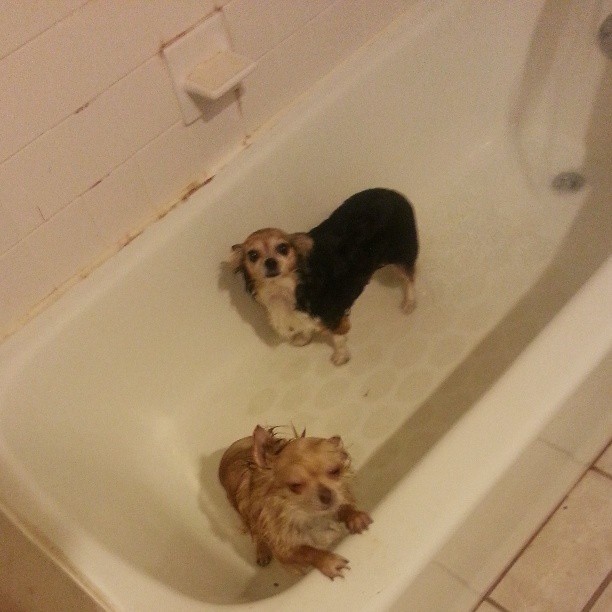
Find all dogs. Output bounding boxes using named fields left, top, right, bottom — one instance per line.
left=218, top=423, right=373, bottom=583
left=231, top=188, right=419, bottom=366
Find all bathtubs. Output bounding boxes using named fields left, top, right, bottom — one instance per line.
left=1, top=1, right=611, bottom=611
